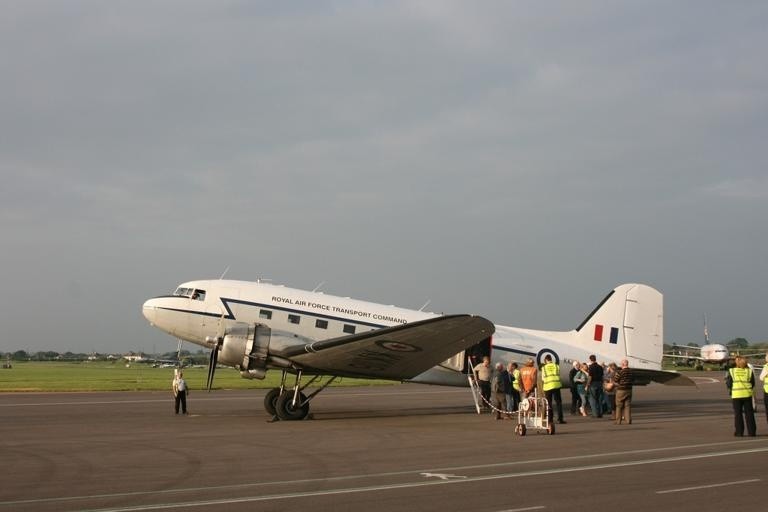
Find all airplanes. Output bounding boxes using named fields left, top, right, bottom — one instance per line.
left=140, top=266, right=702, bottom=424
left=81, top=350, right=208, bottom=370
left=661, top=313, right=767, bottom=372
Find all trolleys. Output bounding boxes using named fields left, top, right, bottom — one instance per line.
left=516, top=387, right=555, bottom=435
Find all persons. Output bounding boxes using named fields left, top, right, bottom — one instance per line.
left=172, top=368, right=189, bottom=414
left=758, top=353, right=767, bottom=423
left=725, top=358, right=756, bottom=437
left=735, top=356, right=755, bottom=413
left=473, top=354, right=633, bottom=426
